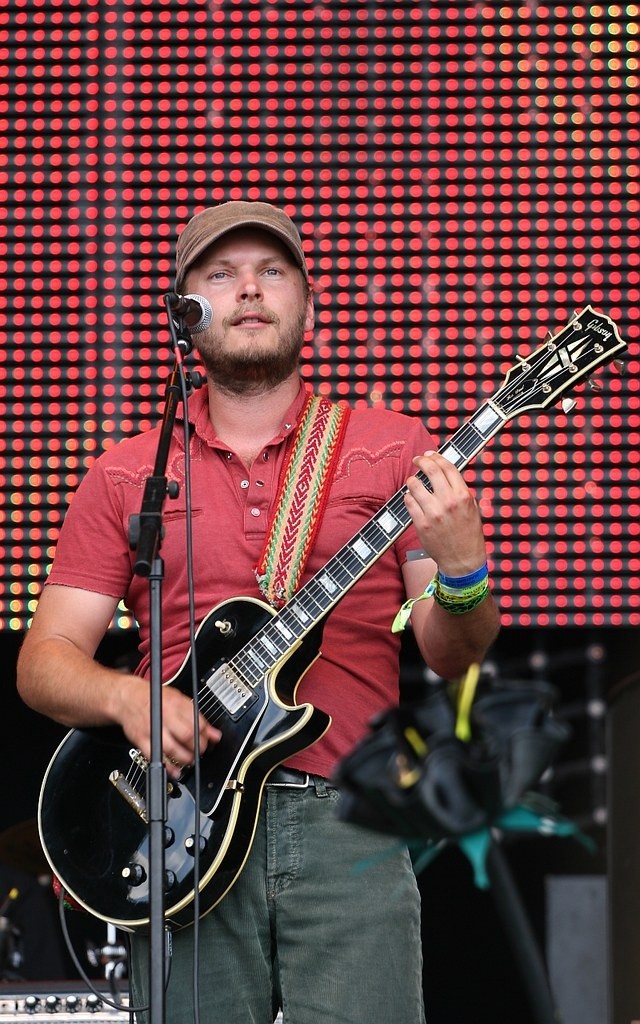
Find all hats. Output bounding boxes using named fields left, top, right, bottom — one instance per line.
left=171, top=200, right=313, bottom=292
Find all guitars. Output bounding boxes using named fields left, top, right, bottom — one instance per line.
left=33, top=296, right=625, bottom=940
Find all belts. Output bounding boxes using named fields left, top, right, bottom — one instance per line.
left=261, top=766, right=342, bottom=789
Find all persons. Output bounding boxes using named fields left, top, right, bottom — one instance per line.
left=17, top=199, right=501, bottom=1024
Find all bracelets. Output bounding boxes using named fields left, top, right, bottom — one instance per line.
left=391, top=564, right=491, bottom=633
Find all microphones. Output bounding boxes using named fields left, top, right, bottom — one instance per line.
left=164, top=292, right=214, bottom=334
